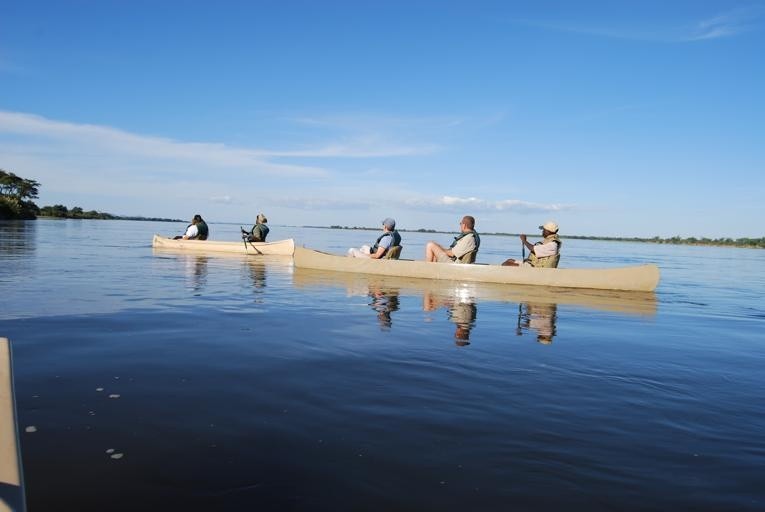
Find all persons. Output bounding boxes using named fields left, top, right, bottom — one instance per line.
left=523, top=302, right=558, bottom=344
left=347, top=216, right=401, bottom=261
left=173, top=213, right=210, bottom=241
left=242, top=213, right=270, bottom=242
left=369, top=282, right=401, bottom=333
left=502, top=219, right=562, bottom=269
left=422, top=294, right=478, bottom=347
left=425, top=215, right=480, bottom=263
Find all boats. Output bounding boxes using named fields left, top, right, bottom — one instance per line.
left=150, top=232, right=296, bottom=259
left=292, top=270, right=659, bottom=317
left=289, top=247, right=662, bottom=294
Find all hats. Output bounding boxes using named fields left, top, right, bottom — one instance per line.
left=539, top=220, right=560, bottom=234
left=381, top=217, right=396, bottom=230
